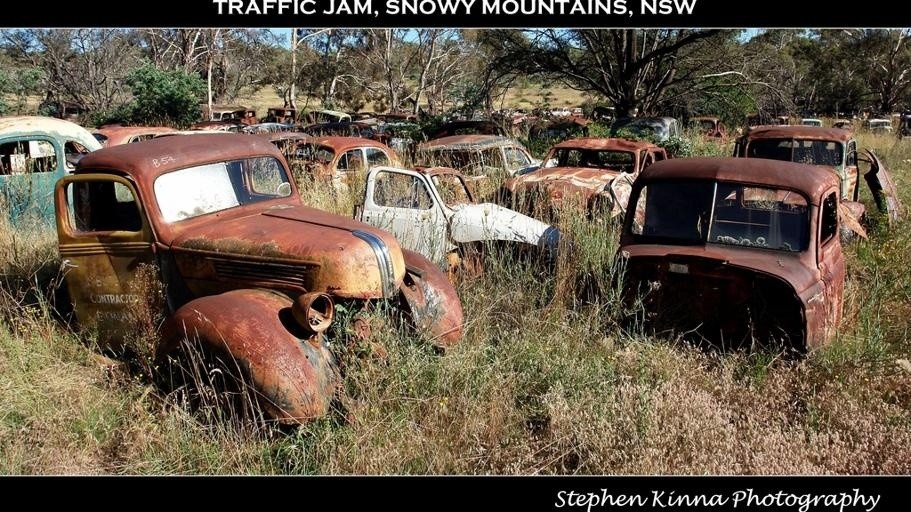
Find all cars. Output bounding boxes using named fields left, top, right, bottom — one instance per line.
left=733, top=107, right=895, bottom=138
